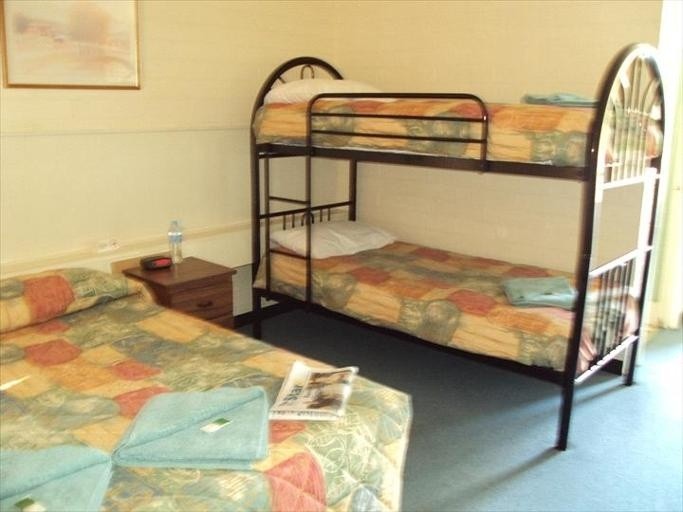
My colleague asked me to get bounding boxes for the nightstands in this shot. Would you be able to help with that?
[121,256,237,331]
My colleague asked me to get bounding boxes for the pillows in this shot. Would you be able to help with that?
[263,78,398,103]
[0,267,143,333]
[269,219,396,259]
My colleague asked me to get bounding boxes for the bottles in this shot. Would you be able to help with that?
[166,217,184,264]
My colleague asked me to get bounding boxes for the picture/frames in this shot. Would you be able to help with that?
[0,0,141,91]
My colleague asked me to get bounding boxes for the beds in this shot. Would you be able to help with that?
[252,42,672,450]
[0,268,414,512]
